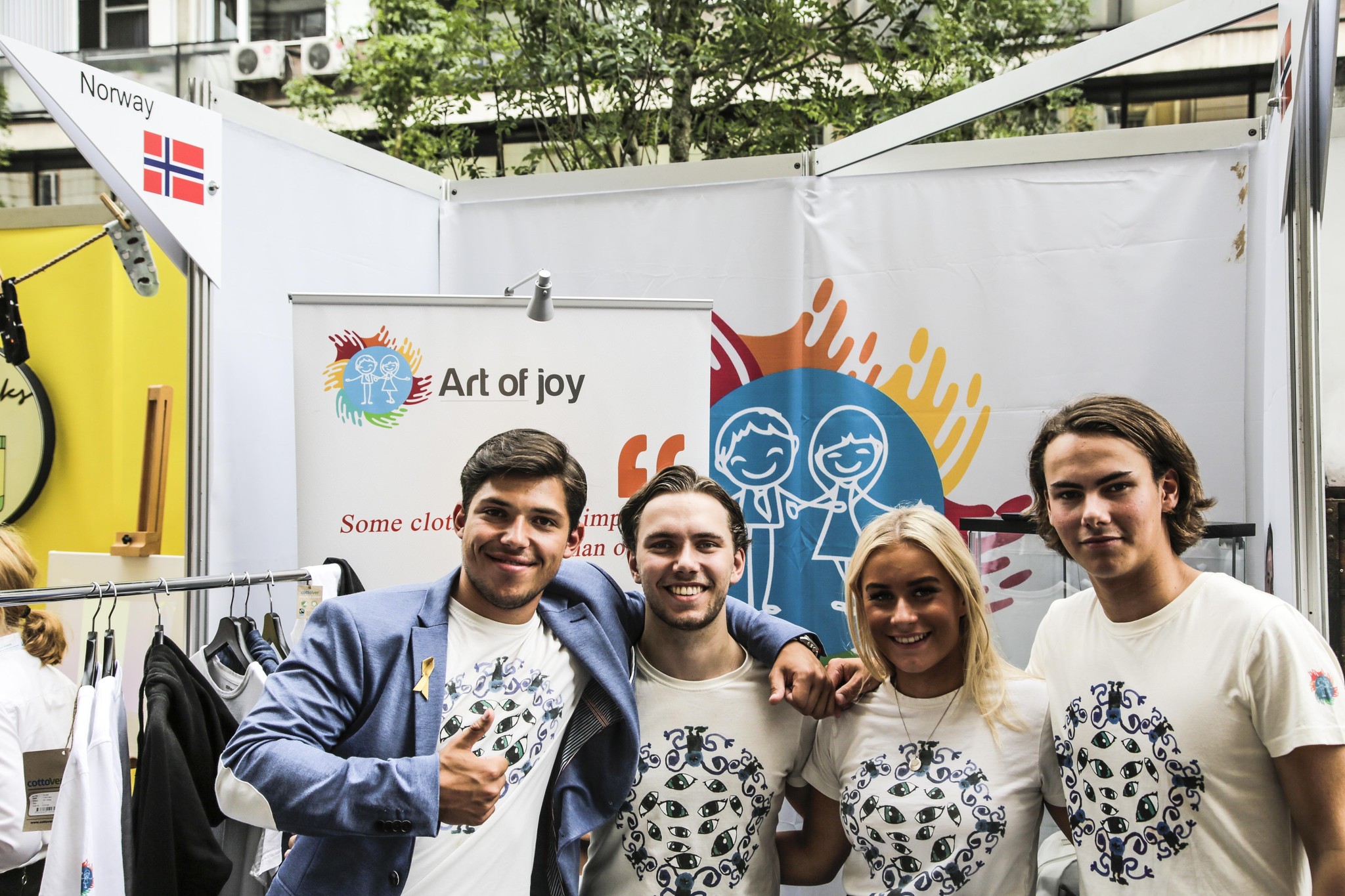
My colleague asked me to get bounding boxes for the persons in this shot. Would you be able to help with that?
[809,391,1345,896]
[0,517,93,896]
[763,503,1094,896]
[578,462,857,896]
[214,427,834,896]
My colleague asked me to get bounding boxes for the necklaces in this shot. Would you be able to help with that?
[888,668,977,774]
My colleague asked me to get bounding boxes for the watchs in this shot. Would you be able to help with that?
[779,629,822,661]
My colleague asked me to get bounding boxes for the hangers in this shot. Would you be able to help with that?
[80,581,104,690]
[236,571,257,639]
[98,581,119,681]
[202,571,255,671]
[152,576,170,646]
[259,569,292,660]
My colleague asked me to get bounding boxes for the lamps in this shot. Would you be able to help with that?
[503,268,556,322]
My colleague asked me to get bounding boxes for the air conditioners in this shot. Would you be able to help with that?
[299,34,356,76]
[229,38,286,82]
